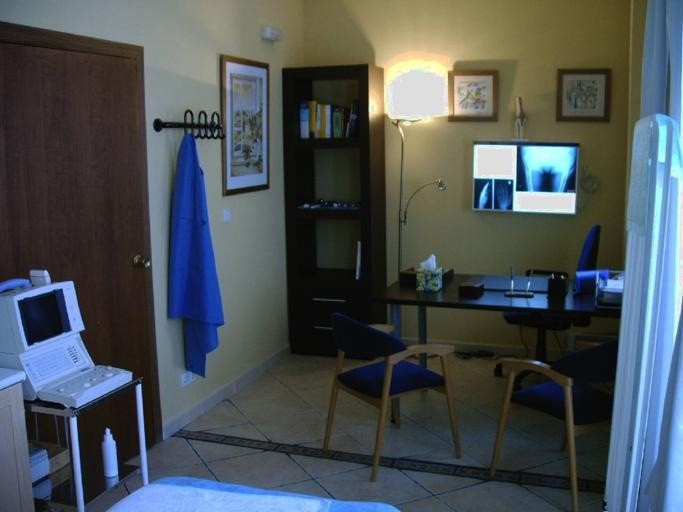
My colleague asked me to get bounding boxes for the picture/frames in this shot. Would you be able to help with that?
[557,68,611,122]
[447,71,498,122]
[220,52,269,197]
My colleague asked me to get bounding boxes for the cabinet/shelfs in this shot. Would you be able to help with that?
[0,366,35,512]
[281,65,386,359]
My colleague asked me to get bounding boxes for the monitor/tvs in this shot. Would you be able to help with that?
[14,280,92,352]
[472,141,580,217]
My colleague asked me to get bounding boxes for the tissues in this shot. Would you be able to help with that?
[416,253,443,295]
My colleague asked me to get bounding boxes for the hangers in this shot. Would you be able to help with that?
[152,109,223,140]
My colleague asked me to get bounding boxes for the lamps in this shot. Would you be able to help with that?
[384,57,448,359]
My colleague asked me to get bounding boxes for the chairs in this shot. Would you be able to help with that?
[323,311,462,483]
[493,225,602,377]
[491,338,618,512]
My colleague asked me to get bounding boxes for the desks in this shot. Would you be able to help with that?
[373,273,625,393]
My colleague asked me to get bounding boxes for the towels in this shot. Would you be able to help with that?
[167,133,226,379]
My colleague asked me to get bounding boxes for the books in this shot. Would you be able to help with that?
[300,100,356,139]
